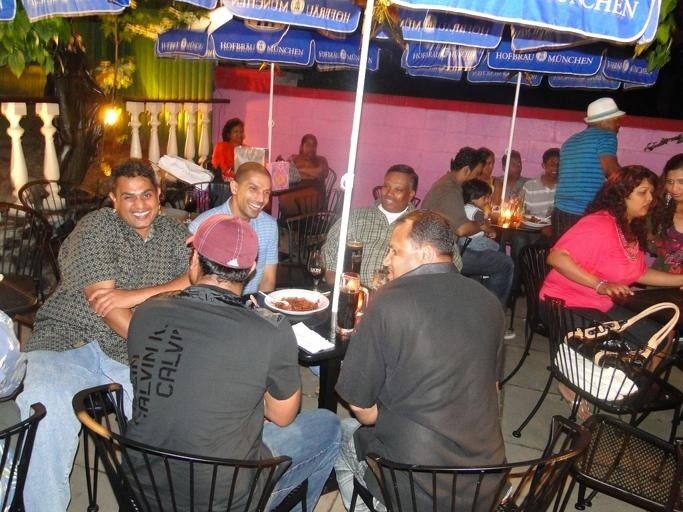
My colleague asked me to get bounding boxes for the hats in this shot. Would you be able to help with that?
[184,212,260,270]
[582,95,627,123]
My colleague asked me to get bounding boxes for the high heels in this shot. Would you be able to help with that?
[556,382,595,422]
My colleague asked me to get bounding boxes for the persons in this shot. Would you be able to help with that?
[0,311,27,509]
[211,118,245,180]
[540,154,682,417]
[424,144,560,338]
[289,134,329,250]
[124,217,341,512]
[322,164,463,288]
[13,158,192,509]
[189,160,280,296]
[333,209,509,512]
[550,98,626,242]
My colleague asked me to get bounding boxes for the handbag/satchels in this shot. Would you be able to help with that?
[233,145,267,174]
[267,160,293,193]
[561,299,683,380]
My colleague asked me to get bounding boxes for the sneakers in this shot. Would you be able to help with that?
[502,328,516,340]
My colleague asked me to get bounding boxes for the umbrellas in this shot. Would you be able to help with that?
[397,42,660,202]
[181,1,664,314]
[153,6,382,164]
[0,0,131,24]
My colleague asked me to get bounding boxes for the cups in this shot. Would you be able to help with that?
[371,270,393,297]
[306,249,327,294]
[342,239,363,275]
[336,272,361,334]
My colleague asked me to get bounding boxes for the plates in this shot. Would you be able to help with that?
[264,288,330,315]
[521,214,552,228]
